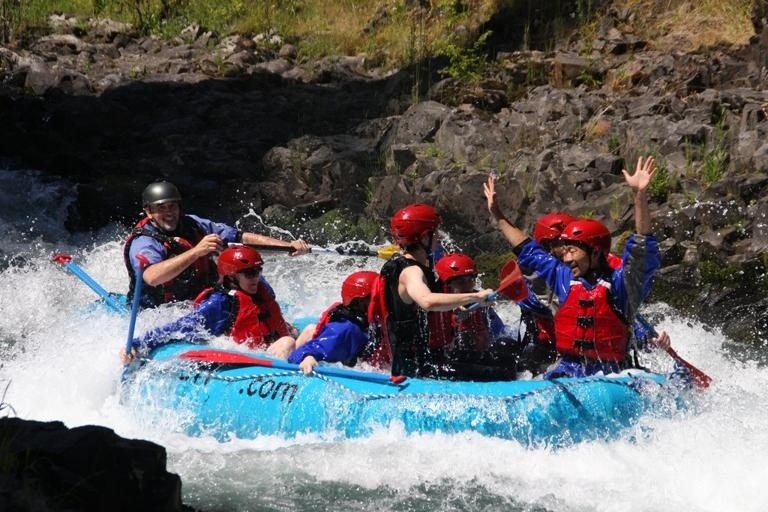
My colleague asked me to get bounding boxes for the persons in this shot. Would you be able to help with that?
[432,250,516,361]
[122,179,312,314]
[482,154,663,380]
[284,269,394,377]
[366,202,520,383]
[117,245,320,365]
[485,212,672,377]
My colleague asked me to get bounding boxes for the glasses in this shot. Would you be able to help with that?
[239,268,261,277]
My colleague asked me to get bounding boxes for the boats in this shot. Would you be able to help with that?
[50,294,687,450]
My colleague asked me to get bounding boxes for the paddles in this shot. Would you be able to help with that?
[457,260,529,321]
[180,351,407,384]
[636,313,714,390]
[220,243,402,262]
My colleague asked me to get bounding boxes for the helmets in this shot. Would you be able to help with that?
[391,204,440,247]
[142,181,184,217]
[535,213,611,269]
[341,271,379,306]
[435,254,477,292]
[216,245,263,284]
